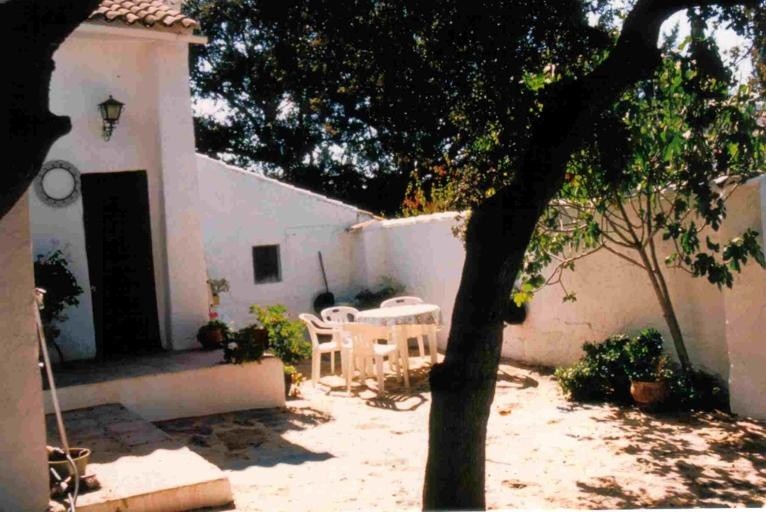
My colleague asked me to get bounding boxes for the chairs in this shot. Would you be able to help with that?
[298,296,438,398]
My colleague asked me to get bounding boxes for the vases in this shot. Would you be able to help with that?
[46,446,90,475]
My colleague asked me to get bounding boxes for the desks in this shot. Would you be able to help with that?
[357,304,440,382]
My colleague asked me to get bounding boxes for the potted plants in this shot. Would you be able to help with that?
[195,319,274,365]
[554,327,666,408]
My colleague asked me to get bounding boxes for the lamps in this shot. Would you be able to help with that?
[95,95,125,141]
